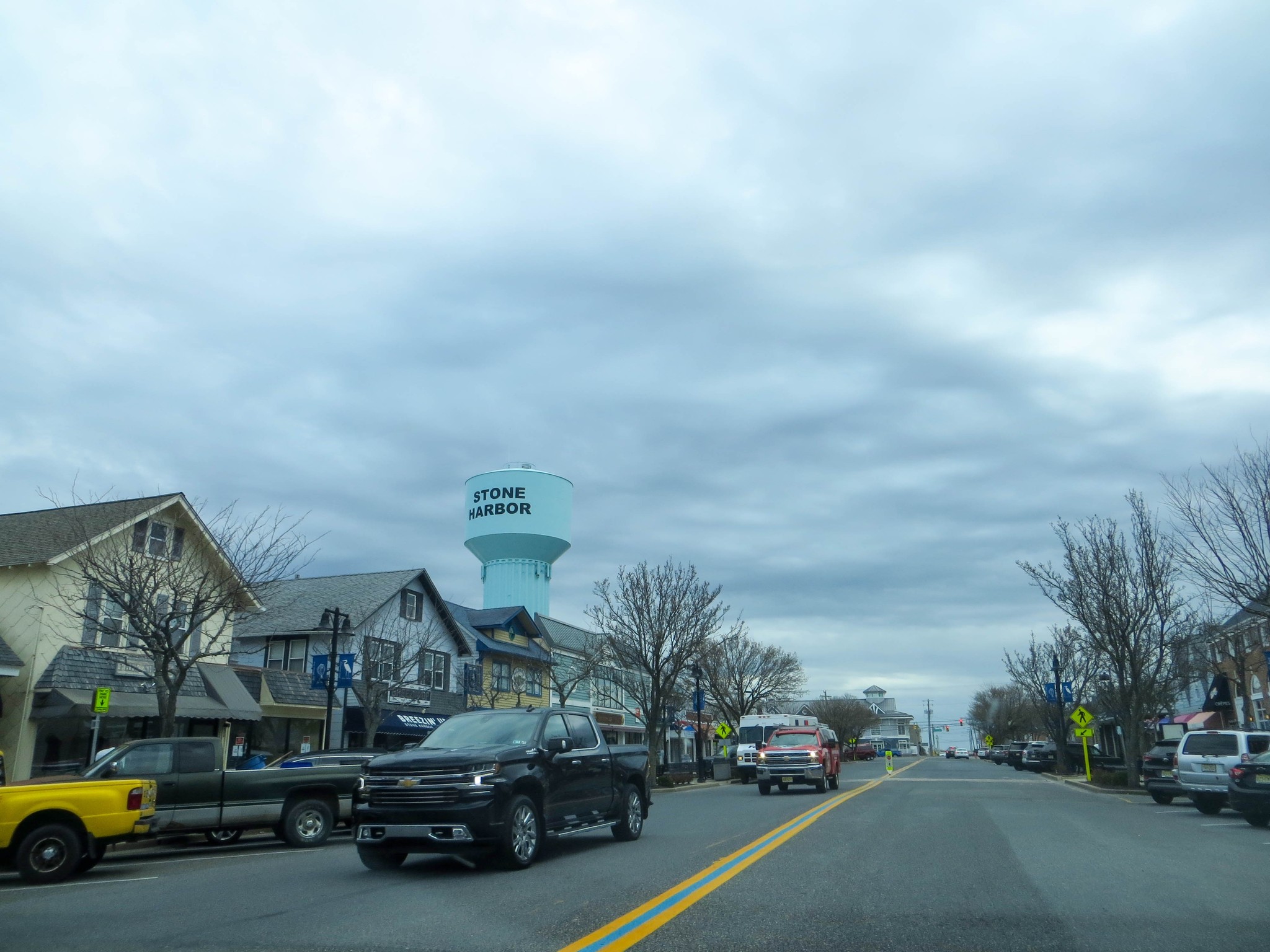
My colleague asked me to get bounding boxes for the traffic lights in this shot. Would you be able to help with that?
[960,719,963,726]
[946,726,949,732]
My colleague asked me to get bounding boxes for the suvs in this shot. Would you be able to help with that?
[977,747,990,760]
[1007,741,1058,774]
[946,746,959,758]
[1142,738,1198,805]
[990,745,1011,765]
[1171,728,1270,815]
[1040,740,1124,774]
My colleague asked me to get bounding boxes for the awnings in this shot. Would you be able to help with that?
[196,661,263,721]
[1202,672,1234,712]
[29,689,231,719]
[1157,712,1221,730]
[344,705,453,737]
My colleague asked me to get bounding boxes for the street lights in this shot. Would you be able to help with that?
[312,606,356,750]
[688,661,708,783]
[1049,649,1067,745]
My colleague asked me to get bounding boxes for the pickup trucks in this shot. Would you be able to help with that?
[875,749,903,757]
[754,723,841,795]
[72,736,364,886]
[350,705,653,873]
[0,745,157,888]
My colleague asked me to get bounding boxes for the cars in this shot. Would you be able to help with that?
[204,748,394,846]
[967,748,979,756]
[954,748,969,759]
[939,752,946,757]
[843,745,877,761]
[1228,749,1270,826]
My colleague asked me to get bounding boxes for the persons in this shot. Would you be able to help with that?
[1136,755,1144,777]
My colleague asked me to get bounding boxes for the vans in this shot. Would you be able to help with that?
[733,713,818,784]
